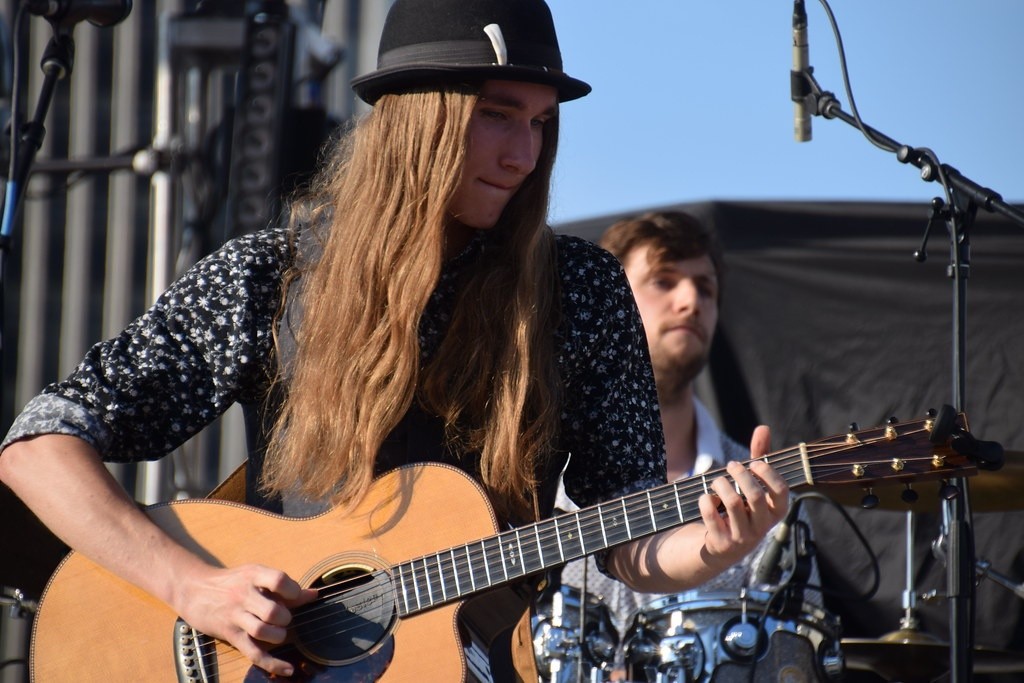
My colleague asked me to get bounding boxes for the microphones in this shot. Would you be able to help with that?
[792,0,814,143]
[21,0,134,28]
[757,499,804,585]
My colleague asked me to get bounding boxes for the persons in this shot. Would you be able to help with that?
[0,1,790,682]
[499,209,845,683]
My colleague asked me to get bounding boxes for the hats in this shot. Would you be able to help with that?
[350,0,593,103]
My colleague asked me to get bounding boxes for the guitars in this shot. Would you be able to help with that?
[28,403,1005,683]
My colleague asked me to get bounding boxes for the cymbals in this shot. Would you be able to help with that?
[784,439,1021,517]
[841,627,1006,682]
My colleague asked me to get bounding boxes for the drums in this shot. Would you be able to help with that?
[530,581,838,683]
